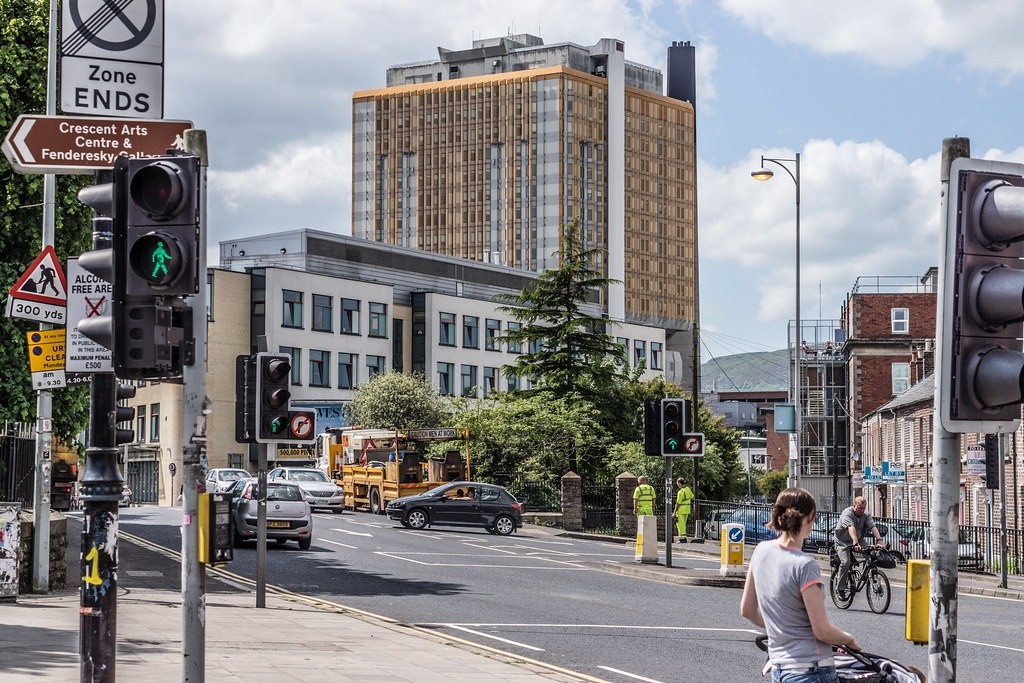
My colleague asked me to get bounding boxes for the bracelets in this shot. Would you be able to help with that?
[877,538,883,541]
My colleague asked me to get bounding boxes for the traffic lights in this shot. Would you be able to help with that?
[256,352,317,444]
[941,158,1024,434]
[116,382,136,447]
[662,399,705,457]
[126,156,198,296]
[978,434,998,489]
[78,156,145,381]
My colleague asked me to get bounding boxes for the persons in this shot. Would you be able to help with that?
[802,340,817,356]
[633,476,657,519]
[740,487,861,683]
[671,478,694,543]
[821,341,832,356]
[448,489,474,501]
[833,496,888,597]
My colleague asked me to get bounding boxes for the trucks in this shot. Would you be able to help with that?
[308,424,470,515]
[50,433,80,511]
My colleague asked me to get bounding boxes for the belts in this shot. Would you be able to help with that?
[762,656,835,676]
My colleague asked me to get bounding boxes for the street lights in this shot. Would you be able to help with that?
[751,153,801,489]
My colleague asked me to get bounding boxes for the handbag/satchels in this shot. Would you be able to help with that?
[870,547,896,569]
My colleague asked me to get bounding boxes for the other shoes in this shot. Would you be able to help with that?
[677,539,687,542]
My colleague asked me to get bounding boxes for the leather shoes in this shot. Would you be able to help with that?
[836,589,846,601]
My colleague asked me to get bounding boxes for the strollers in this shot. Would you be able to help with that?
[754,635,927,683]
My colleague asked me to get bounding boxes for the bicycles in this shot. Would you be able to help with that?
[830,541,892,614]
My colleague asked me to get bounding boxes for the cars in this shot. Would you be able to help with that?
[384,482,523,536]
[704,506,914,564]
[267,467,345,514]
[205,468,252,492]
[224,477,312,550]
[908,527,985,574]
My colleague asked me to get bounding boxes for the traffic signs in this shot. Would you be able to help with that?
[6,113,194,169]
